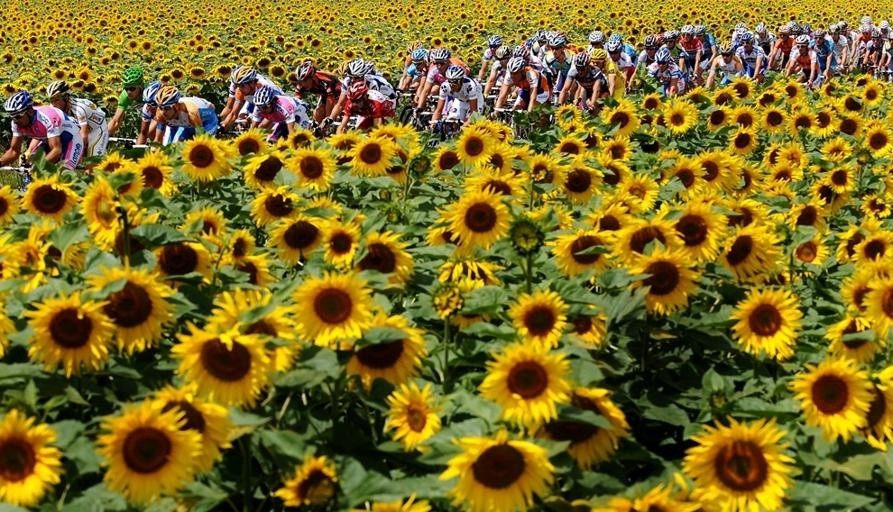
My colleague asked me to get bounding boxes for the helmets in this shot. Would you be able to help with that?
[4,92,33,111]
[407,42,464,84]
[296,61,313,82]
[253,85,273,107]
[155,85,180,106]
[143,83,162,103]
[46,81,68,99]
[337,60,374,103]
[121,67,144,87]
[231,65,256,85]
[488,17,893,73]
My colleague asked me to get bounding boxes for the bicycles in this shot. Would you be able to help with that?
[0,165,39,189]
[397,86,524,130]
[683,70,701,85]
[551,91,599,114]
[106,135,138,145]
[225,116,363,136]
[131,142,159,151]
[782,64,891,88]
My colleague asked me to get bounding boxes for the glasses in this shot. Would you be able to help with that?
[9,112,26,120]
[159,104,176,110]
[124,87,136,91]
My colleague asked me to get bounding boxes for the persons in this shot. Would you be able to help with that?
[396,47,487,127]
[2,92,83,170]
[295,59,399,133]
[477,13,892,110]
[108,66,221,149]
[217,64,321,142]
[47,80,109,156]
